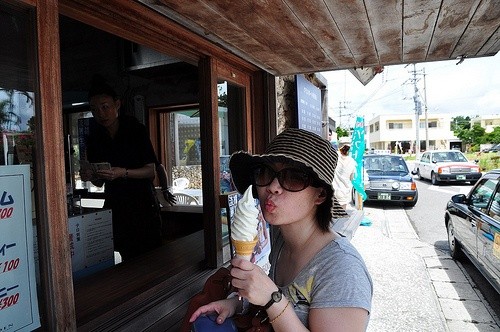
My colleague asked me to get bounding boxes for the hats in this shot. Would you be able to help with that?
[228,127,348,219]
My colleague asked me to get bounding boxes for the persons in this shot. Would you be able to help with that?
[79,82,161,249]
[190,128,373,332]
[391,160,403,170]
[332,136,357,205]
[156,163,177,206]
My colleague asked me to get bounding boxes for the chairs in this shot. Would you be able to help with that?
[172,177,200,205]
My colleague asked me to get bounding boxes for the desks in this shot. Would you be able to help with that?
[178,189,202,199]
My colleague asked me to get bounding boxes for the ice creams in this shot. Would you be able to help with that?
[230,185,259,258]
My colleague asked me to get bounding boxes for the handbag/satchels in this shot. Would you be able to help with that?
[235,303,271,332]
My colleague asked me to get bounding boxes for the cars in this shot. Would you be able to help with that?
[418,150,482,185]
[352,150,418,207]
[482,142,500,153]
[444,169,500,295]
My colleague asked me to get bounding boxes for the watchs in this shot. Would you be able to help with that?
[262,288,283,309]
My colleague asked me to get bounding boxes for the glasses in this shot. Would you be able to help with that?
[250,164,324,192]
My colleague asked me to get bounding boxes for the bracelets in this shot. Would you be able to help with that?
[270,301,290,323]
[125,168,128,176]
